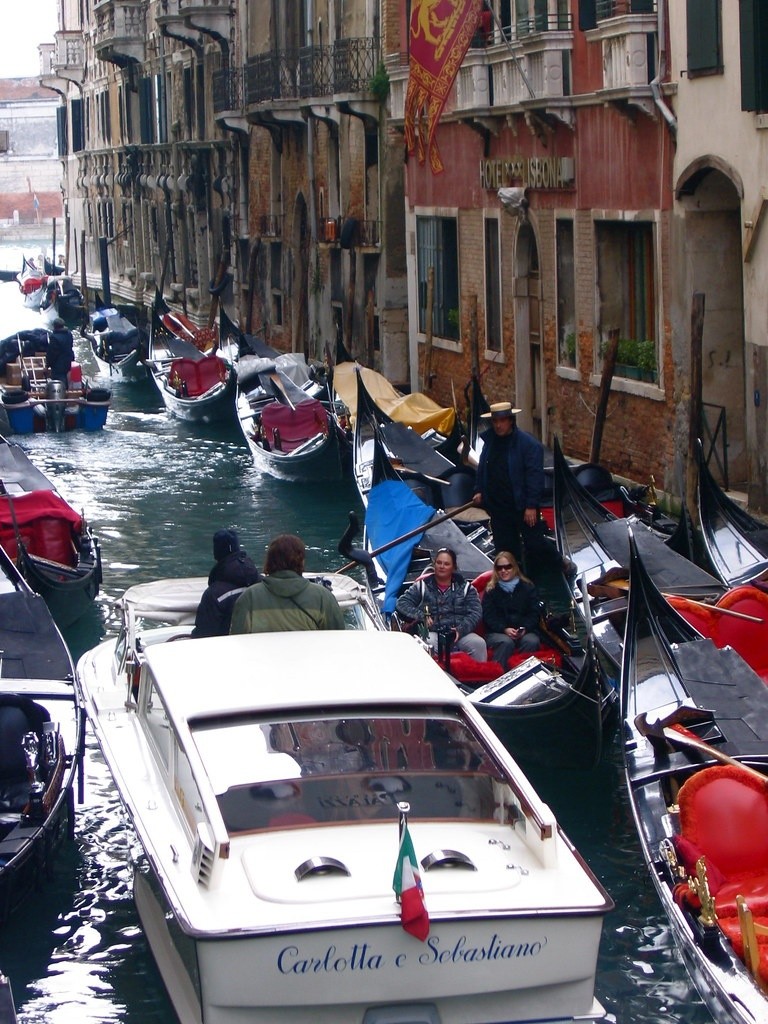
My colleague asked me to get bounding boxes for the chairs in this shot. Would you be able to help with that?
[714,585,768,689]
[666,595,713,639]
[261,398,329,452]
[540,472,556,510]
[412,569,567,679]
[0,517,74,580]
[575,463,623,503]
[169,354,227,397]
[669,765,768,1002]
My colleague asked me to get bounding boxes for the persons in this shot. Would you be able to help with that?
[43,317,75,391]
[190,531,265,640]
[396,548,488,663]
[29,255,66,271]
[473,402,544,588]
[0,693,51,813]
[481,552,540,673]
[228,535,345,636]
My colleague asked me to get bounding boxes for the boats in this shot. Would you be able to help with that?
[0,328,113,435]
[219,300,337,402]
[324,325,455,446]
[38,274,86,329]
[0,431,104,601]
[42,255,66,275]
[76,571,617,1024]
[351,364,618,747]
[230,330,348,483]
[88,289,139,376]
[155,286,221,359]
[146,301,233,422]
[551,431,768,706]
[617,525,768,1024]
[0,544,87,909]
[15,255,47,296]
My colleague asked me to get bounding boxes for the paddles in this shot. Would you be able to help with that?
[167,314,196,339]
[135,356,184,367]
[270,374,296,412]
[334,500,476,574]
[662,726,768,783]
[391,463,451,485]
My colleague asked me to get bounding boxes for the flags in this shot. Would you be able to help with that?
[392,821,431,944]
[33,191,39,210]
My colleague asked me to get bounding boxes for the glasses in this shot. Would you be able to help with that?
[495,563,513,571]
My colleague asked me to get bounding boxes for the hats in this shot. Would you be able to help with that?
[213,530,241,559]
[480,402,523,419]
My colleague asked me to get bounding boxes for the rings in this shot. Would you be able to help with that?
[509,634,512,636]
